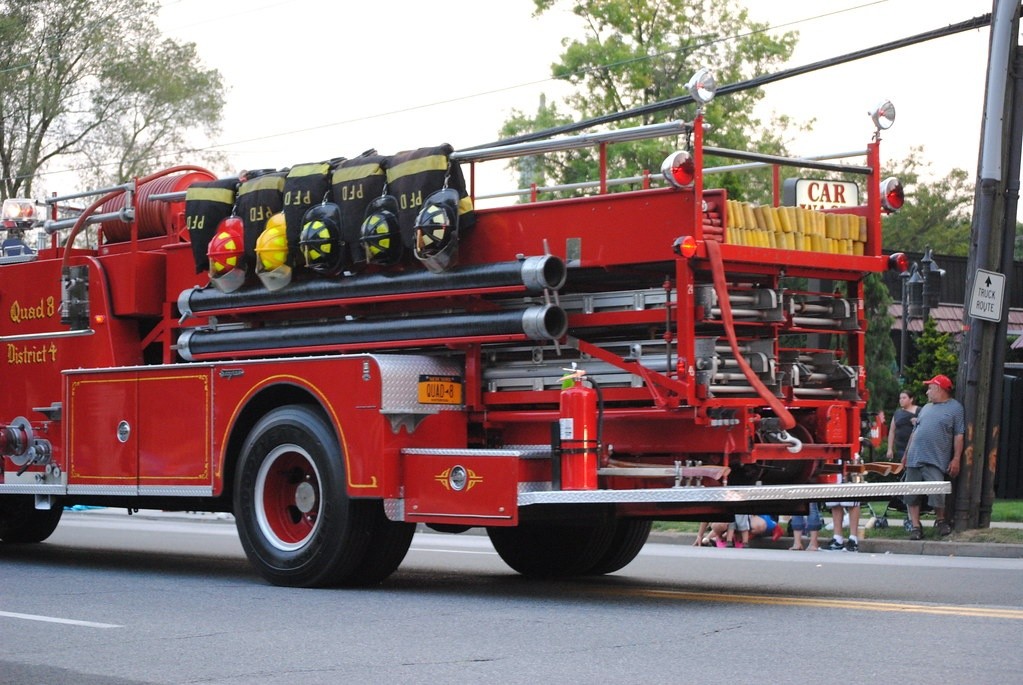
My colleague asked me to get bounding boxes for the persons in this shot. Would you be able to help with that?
[900,375,965,541]
[886,390,922,481]
[692,452,860,553]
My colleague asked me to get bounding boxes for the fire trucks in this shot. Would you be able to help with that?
[0,67,954,591]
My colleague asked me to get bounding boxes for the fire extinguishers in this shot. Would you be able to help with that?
[557,361,604,490]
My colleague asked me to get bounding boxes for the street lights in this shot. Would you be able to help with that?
[897,261,923,377]
[920,244,946,333]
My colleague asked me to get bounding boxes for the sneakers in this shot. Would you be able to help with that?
[841,539,859,553]
[909,521,925,540]
[934,519,951,537]
[817,538,847,552]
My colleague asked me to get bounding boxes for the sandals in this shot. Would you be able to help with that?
[805,543,820,552]
[788,545,805,550]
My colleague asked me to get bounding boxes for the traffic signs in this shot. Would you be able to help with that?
[968,268,1007,323]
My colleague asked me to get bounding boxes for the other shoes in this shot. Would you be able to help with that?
[771,525,784,541]
[748,532,758,540]
[742,542,751,548]
[726,541,733,548]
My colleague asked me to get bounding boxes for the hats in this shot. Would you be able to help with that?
[922,374,952,392]
